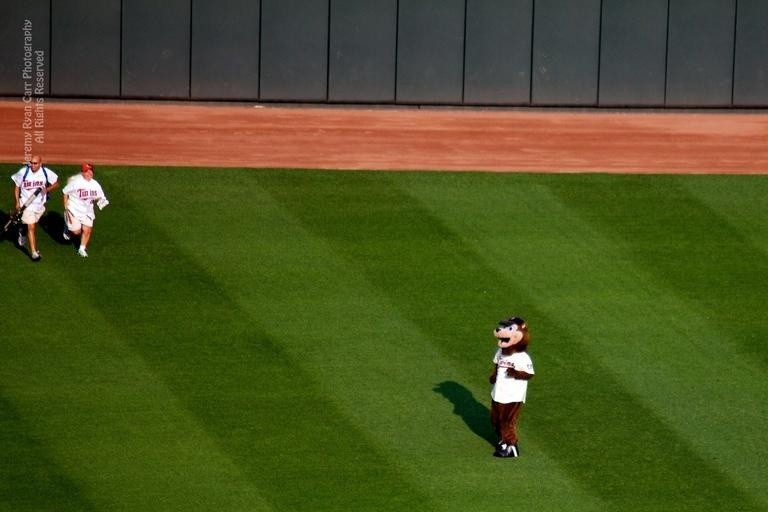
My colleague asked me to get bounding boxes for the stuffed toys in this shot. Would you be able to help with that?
[490,316,536,458]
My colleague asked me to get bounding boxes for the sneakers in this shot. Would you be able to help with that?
[18,231,26,246]
[32,251,40,260]
[76,248,89,258]
[63,226,71,241]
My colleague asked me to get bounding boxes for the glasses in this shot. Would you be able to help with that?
[29,161,38,164]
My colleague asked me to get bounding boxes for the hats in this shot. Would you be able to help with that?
[81,163,91,172]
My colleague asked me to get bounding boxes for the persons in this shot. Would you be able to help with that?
[61,162,109,258]
[10,155,60,262]
